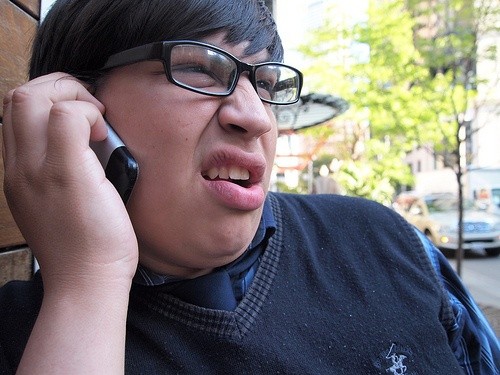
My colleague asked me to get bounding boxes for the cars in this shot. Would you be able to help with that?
[406,193,500,258]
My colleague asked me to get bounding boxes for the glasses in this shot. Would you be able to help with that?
[90,40,303,106]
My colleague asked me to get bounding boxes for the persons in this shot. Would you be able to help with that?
[0,0,500,375]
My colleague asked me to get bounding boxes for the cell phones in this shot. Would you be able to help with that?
[89,117,139,208]
